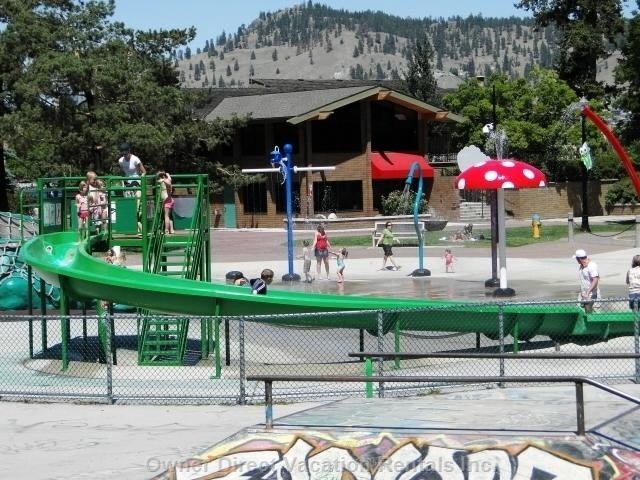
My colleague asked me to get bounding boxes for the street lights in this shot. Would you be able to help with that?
[577,95,593,233]
[475,74,497,159]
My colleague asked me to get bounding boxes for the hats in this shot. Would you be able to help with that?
[573,250,586,258]
[120,143,129,155]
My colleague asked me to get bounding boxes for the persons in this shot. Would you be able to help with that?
[296,240,316,283]
[250,267,274,296]
[441,247,459,273]
[571,249,603,313]
[373,220,405,272]
[625,254,640,312]
[118,142,146,183]
[328,247,349,284]
[104,249,117,264]
[75,169,175,236]
[113,252,126,267]
[311,223,334,281]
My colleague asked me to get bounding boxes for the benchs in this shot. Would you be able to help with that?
[371,222,425,249]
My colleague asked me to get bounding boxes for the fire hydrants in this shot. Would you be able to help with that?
[531,213,543,239]
